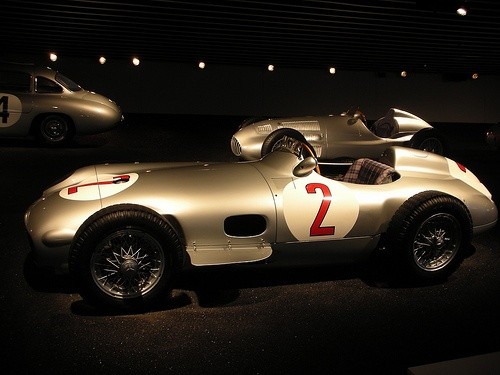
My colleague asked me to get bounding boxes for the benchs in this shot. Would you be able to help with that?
[343,157,395,186]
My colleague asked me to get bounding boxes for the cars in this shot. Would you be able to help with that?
[2,62,127,149]
[25,149,498,320]
[230,106,447,170]
